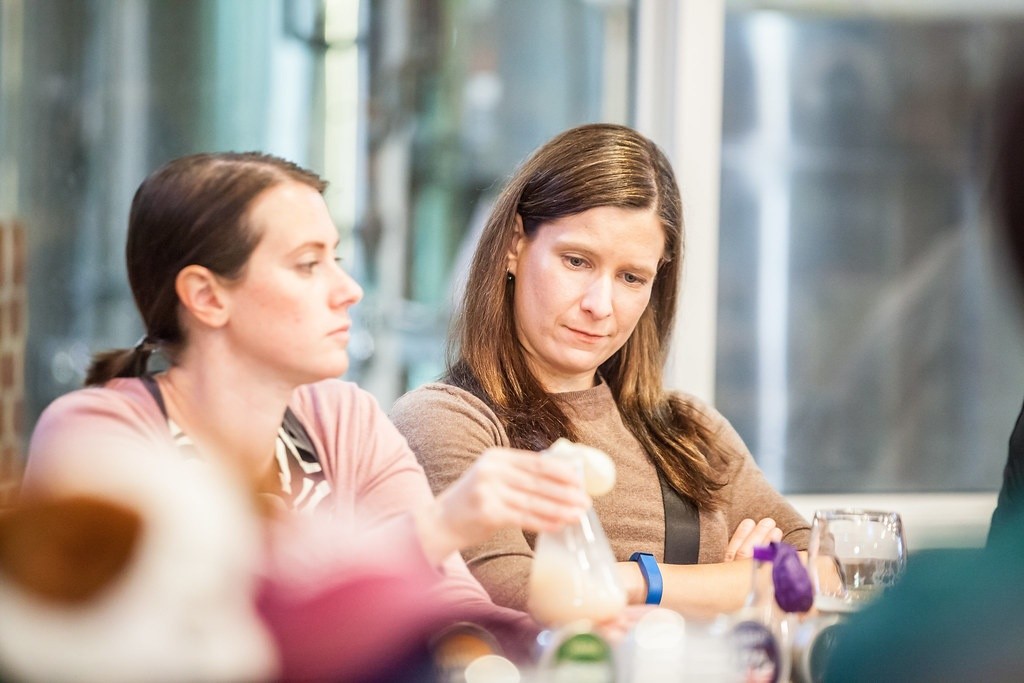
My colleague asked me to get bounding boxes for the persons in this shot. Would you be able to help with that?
[773,34,1024,682]
[11,150,589,683]
[387,121,845,628]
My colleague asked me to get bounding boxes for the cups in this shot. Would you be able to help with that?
[810,507,909,626]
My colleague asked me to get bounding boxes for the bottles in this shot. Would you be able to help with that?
[523,449,630,624]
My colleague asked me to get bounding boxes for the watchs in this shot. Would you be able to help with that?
[629,552,662,605]
